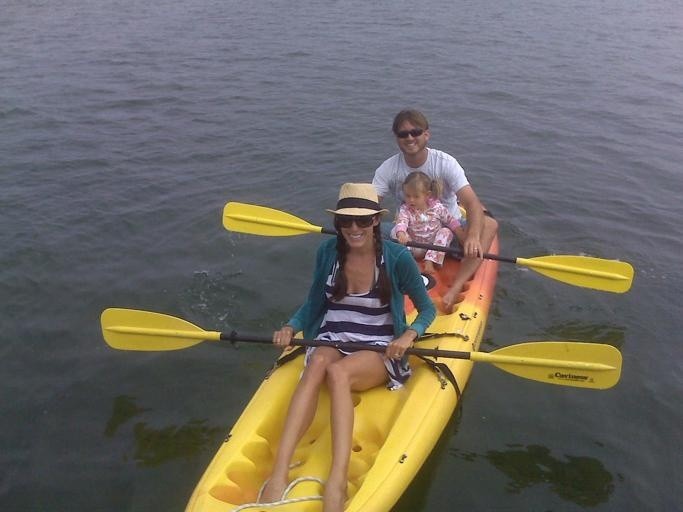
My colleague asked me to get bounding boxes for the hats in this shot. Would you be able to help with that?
[325,183,390,216]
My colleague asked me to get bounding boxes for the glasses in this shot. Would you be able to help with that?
[397,128,423,138]
[336,216,373,228]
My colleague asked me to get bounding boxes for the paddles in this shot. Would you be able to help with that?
[221,201,634,294]
[100,308,622,391]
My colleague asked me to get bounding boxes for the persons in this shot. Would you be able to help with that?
[389,170,468,276]
[257,181,436,512]
[371,109,498,315]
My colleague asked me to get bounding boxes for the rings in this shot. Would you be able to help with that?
[393,354,398,356]
[473,248,477,250]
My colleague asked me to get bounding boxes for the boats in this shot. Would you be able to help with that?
[182,200,500,512]
